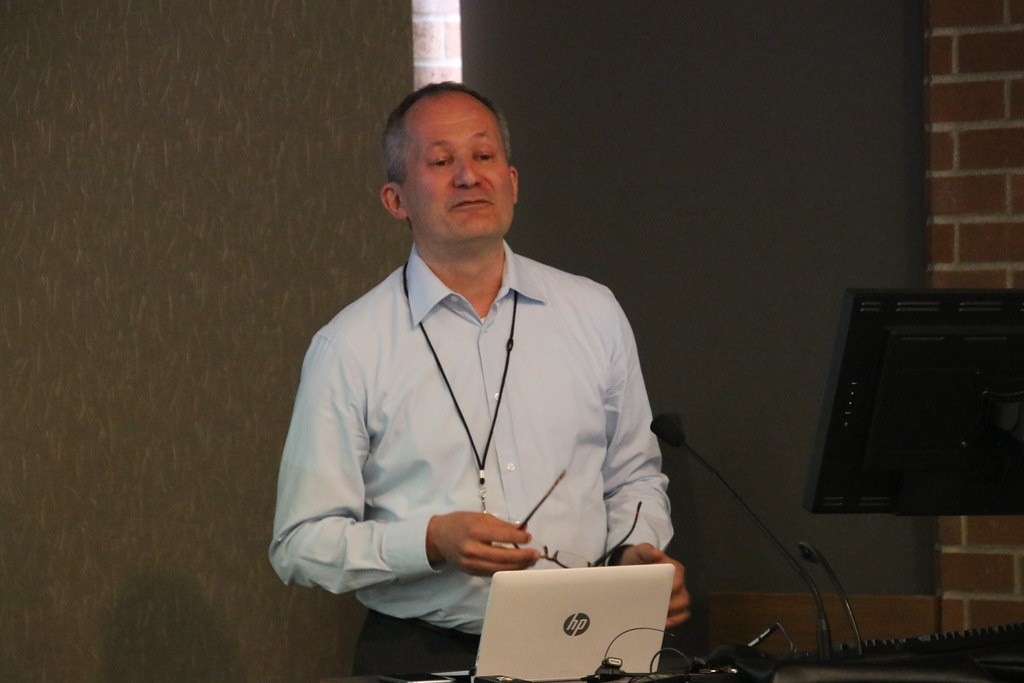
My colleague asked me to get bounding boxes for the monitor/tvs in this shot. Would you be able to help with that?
[803,286,1024,516]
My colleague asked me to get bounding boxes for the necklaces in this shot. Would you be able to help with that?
[398,259,518,512]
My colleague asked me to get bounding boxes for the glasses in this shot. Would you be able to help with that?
[514,470,643,568]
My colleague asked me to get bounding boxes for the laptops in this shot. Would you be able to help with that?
[429,564,675,683]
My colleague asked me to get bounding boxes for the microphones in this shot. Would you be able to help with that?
[650,413,831,662]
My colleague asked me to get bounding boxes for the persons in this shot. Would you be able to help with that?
[268,82,692,682]
